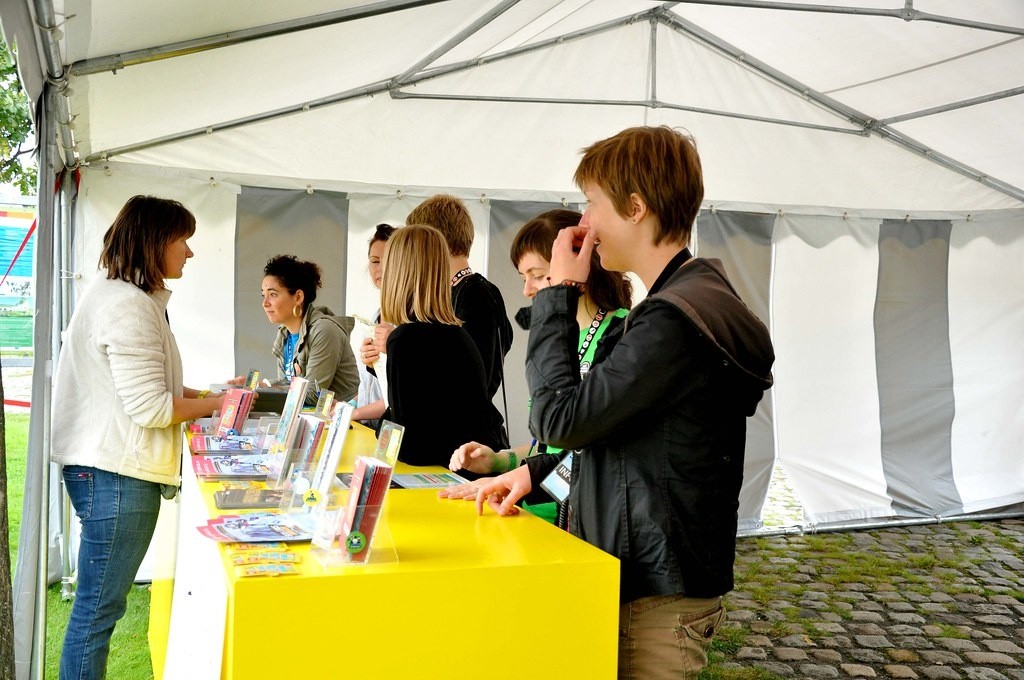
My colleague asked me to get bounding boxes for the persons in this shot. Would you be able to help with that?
[226,255,359,414]
[438,210,629,530]
[473,128,773,680]
[398,194,514,458]
[47,197,259,680]
[352,222,399,426]
[375,224,503,480]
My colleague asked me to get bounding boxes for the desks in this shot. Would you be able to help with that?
[146,408,621,680]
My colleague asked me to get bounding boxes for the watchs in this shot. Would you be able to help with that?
[499,449,517,470]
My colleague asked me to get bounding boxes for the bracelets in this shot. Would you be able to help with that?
[197,390,213,401]
[558,280,586,294]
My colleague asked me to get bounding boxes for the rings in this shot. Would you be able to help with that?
[493,491,498,496]
[362,352,368,358]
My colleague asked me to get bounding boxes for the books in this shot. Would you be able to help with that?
[184,378,393,570]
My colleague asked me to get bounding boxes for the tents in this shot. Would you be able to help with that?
[1,0,1023,680]
